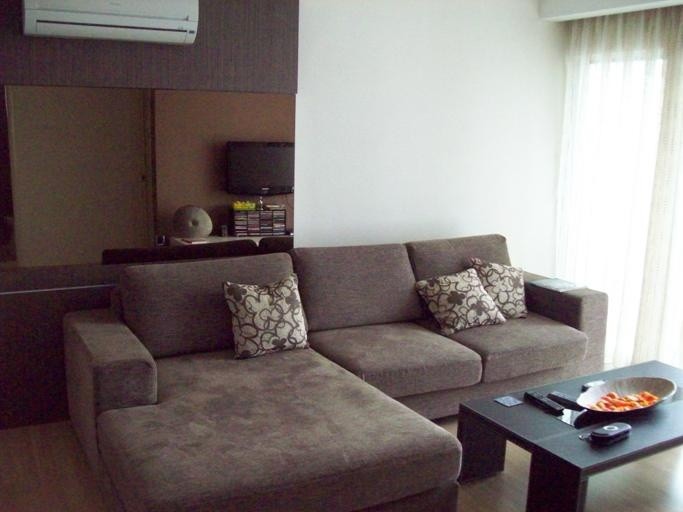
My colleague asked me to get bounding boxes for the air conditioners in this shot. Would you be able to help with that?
[22,0,200,45]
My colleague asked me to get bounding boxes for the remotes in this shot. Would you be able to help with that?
[548,391,586,410]
[526,391,564,413]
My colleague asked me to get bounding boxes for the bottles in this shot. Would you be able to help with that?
[256,196,264,211]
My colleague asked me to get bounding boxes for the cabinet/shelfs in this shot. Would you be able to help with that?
[228,208,286,237]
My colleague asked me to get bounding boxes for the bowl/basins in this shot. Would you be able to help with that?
[574,375,677,413]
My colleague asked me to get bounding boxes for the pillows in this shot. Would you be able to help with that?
[220,272,309,361]
[468,251,527,318]
[415,267,505,335]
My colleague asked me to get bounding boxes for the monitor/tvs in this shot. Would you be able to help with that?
[224,141,294,196]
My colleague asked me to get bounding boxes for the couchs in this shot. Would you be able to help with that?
[61,233,610,512]
